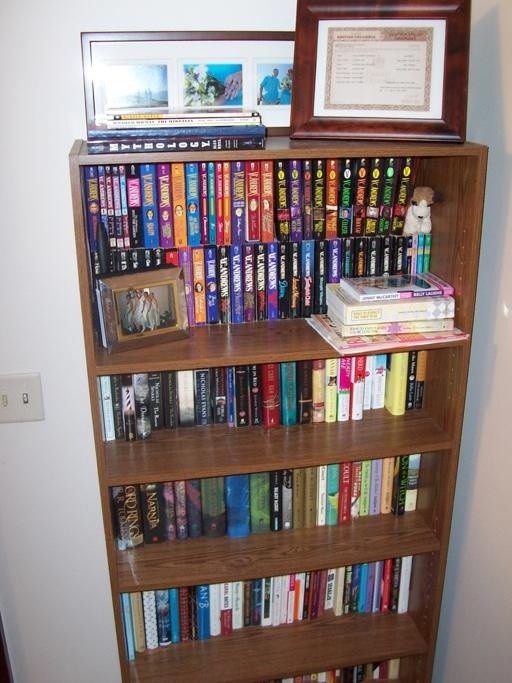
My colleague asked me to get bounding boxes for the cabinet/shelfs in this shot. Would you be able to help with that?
[68,140,488,683]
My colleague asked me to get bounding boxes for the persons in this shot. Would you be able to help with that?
[259,67,292,105]
[184,65,243,105]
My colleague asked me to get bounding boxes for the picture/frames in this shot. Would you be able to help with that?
[289,0,471,143]
[79,31,296,140]
[95,264,191,356]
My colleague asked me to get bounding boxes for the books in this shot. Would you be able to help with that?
[109,454,421,549]
[100,349,427,441]
[84,158,468,354]
[273,658,400,682]
[87,109,267,153]
[122,555,413,660]
